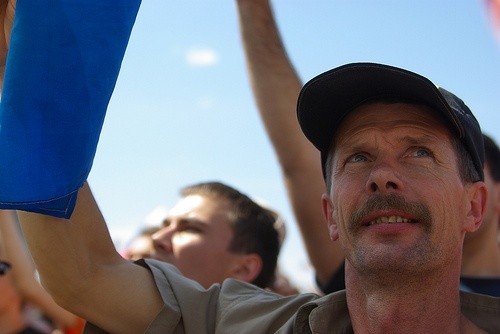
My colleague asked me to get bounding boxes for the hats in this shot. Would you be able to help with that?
[296,63,485,181]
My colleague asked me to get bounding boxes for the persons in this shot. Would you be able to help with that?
[0,257,52,334]
[237,0,500,298]
[0,1,500,334]
[0,209,160,334]
[0,182,279,333]
